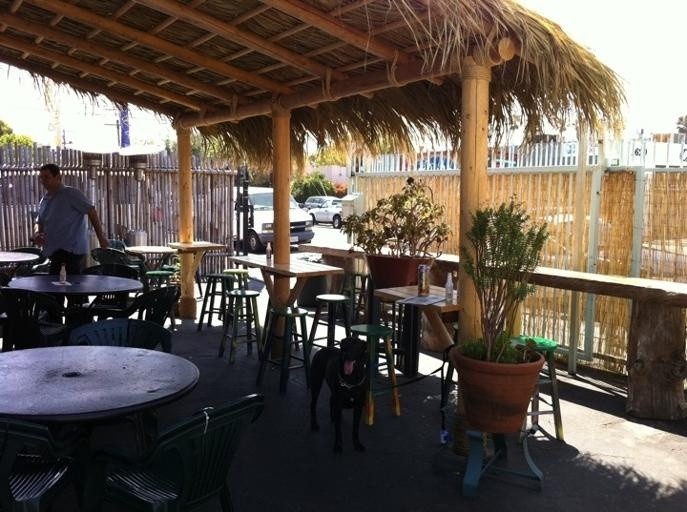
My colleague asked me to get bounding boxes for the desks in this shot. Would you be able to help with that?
[168,241,227,319]
[374,283,461,353]
[0,345,200,458]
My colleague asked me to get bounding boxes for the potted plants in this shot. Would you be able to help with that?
[449,193,546,431]
[340,178,452,302]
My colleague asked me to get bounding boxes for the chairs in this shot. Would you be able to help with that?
[105,391,265,512]
[0,239,181,353]
[0,418,86,512]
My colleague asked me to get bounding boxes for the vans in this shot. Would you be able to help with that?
[204,184,311,253]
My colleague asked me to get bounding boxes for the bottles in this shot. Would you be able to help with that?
[59,262,67,284]
[417,264,430,297]
[265,240,272,259]
[444,272,454,304]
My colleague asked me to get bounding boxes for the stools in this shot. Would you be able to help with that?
[198,269,401,425]
[510,337,564,442]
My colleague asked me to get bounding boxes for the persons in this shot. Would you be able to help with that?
[33,164,110,324]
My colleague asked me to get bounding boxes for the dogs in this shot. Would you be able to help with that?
[309,337,369,457]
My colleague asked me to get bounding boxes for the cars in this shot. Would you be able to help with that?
[304,196,348,225]
